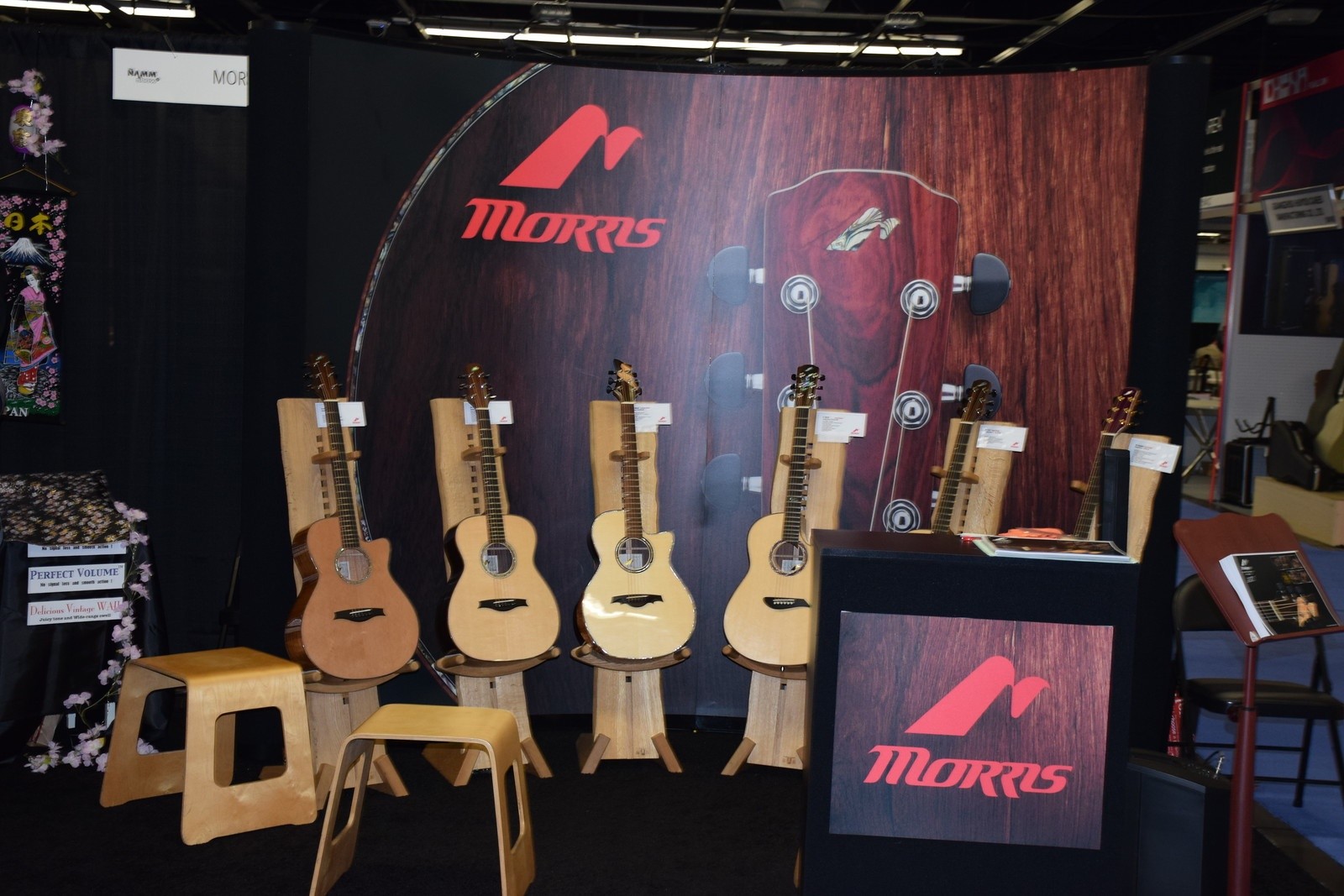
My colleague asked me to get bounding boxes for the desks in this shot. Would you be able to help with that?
[1182,400,1220,482]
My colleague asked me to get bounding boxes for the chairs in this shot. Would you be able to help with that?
[1171,577,1344,806]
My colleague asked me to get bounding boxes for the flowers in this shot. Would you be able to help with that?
[26,502,164,778]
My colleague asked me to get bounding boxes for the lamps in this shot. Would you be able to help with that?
[886,11,927,28]
[531,2,572,21]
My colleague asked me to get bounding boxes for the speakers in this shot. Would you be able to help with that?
[1124,747,1232,896]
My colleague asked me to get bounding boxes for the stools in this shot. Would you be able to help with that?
[100,647,320,847]
[309,704,535,896]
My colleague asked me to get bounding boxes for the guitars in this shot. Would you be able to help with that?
[284,350,421,680]
[576,363,698,665]
[1073,387,1140,540]
[723,364,825,667]
[438,362,562,662]
[931,379,997,534]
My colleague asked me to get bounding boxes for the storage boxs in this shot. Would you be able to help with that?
[1253,476,1344,546]
